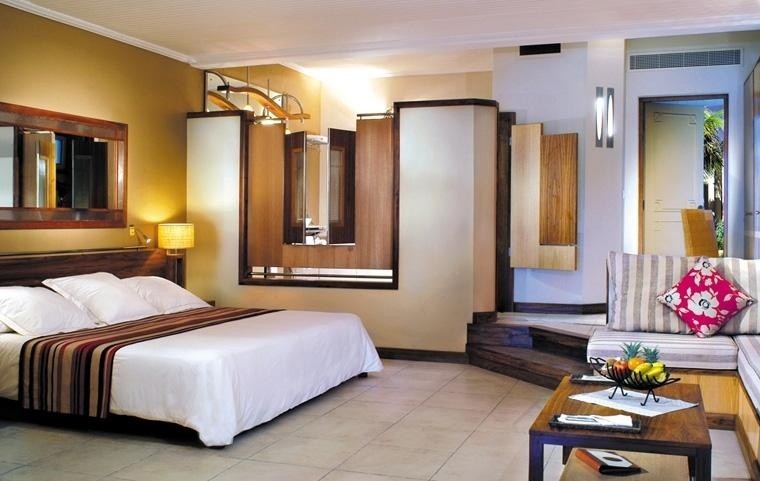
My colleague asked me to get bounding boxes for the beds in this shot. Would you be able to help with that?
[1,249,385,450]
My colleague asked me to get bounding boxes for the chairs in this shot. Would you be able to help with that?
[682,206,719,258]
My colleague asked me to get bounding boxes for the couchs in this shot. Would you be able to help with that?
[586,249,760,480]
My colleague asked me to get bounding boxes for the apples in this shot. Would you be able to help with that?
[613,361,628,375]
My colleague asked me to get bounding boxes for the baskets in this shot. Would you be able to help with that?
[590,355,680,391]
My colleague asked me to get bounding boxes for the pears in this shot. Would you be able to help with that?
[628,357,644,371]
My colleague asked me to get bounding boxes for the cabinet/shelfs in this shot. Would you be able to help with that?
[743,53,760,260]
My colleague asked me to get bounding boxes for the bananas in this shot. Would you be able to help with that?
[633,363,652,375]
[652,363,665,368]
[642,367,665,382]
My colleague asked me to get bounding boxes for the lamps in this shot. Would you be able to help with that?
[593,81,616,151]
[157,222,197,284]
[135,228,151,246]
[239,64,293,137]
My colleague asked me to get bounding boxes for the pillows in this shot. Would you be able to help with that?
[656,255,756,342]
[116,275,214,316]
[1,285,99,337]
[41,270,159,327]
